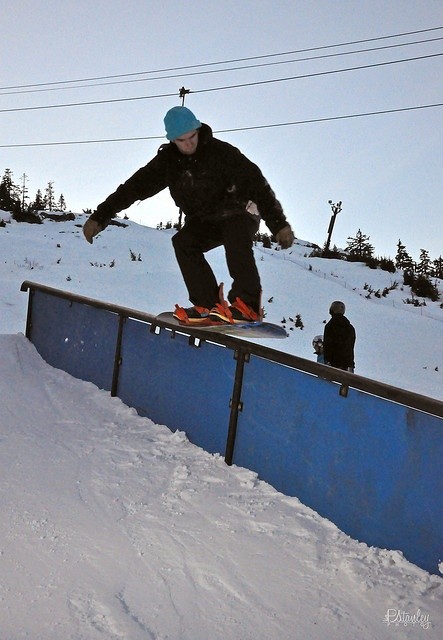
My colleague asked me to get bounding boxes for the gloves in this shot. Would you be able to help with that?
[275,225,296,250]
[82,218,102,244]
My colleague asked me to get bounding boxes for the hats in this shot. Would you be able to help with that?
[164,106,202,140]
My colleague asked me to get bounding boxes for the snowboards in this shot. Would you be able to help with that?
[153,312,287,339]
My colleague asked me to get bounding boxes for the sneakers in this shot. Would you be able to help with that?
[173,305,210,322]
[210,297,257,324]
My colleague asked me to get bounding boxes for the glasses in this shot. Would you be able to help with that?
[312,340,322,349]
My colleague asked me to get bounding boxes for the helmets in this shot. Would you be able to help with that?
[330,301,345,315]
[313,335,324,344]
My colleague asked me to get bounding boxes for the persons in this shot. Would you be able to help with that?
[322,301,356,374]
[84,107,295,326]
[312,335,324,364]
